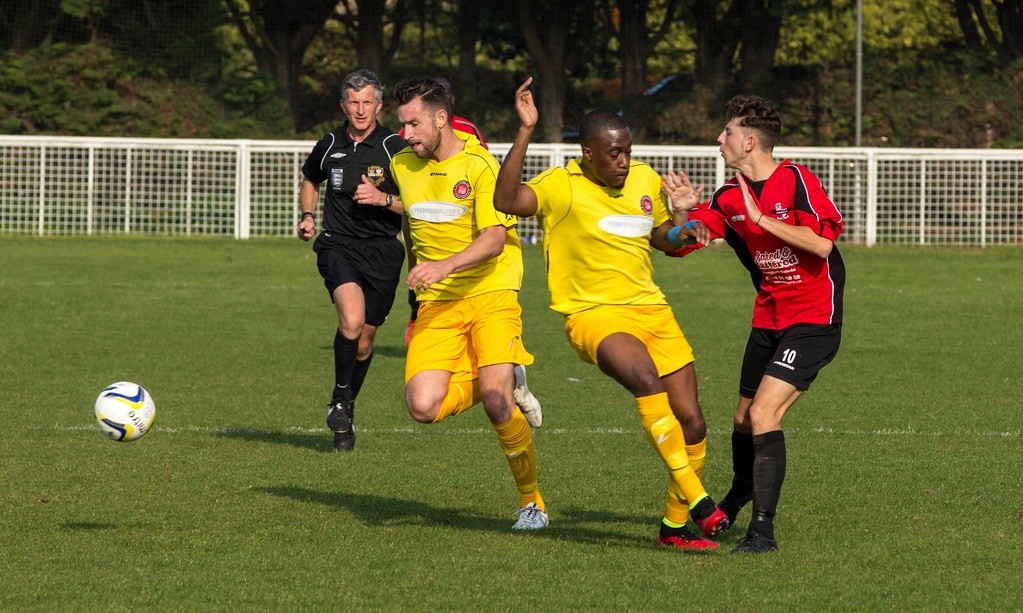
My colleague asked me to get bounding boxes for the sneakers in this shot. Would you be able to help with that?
[511,504,549,530]
[716,501,735,530]
[690,496,729,537]
[326,397,349,433]
[731,532,779,554]
[656,521,719,550]
[334,418,355,451]
[512,363,544,429]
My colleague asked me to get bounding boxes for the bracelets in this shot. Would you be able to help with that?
[302,212,314,219]
[756,214,763,225]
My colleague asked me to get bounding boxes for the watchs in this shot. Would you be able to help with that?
[385,192,394,207]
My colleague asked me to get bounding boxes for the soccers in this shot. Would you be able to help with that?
[94,380,157,443]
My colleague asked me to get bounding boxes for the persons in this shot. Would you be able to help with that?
[388,78,549,531]
[493,77,729,552]
[662,94,846,555]
[297,68,405,452]
[398,77,489,346]
